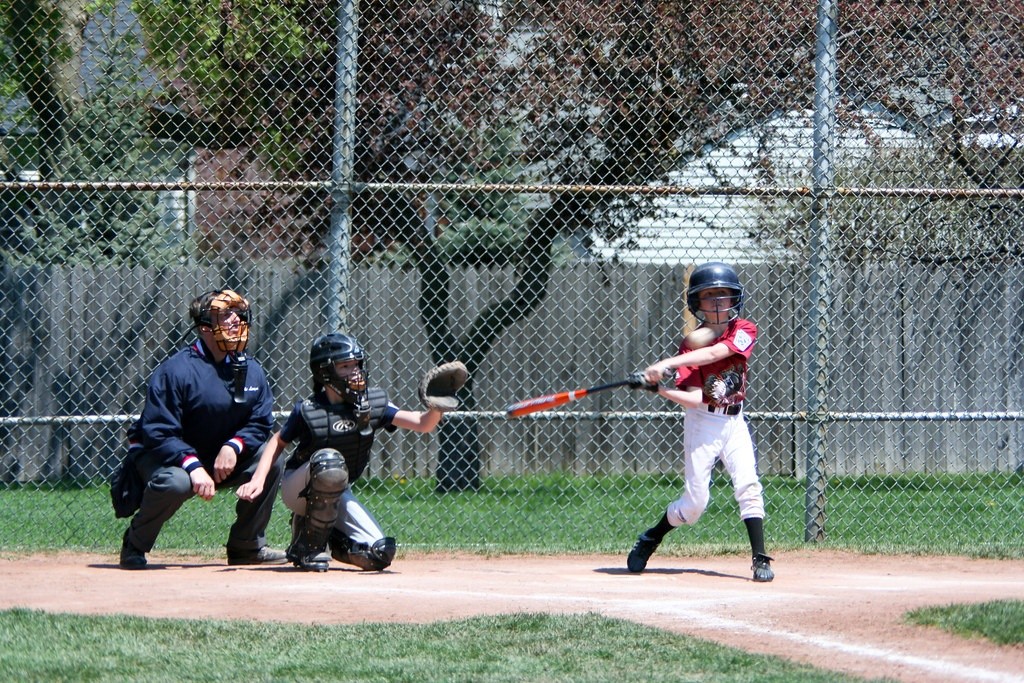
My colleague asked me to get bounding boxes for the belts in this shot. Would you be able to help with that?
[709,403,742,415]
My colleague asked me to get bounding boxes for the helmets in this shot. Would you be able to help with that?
[687,262,745,325]
[310,332,371,405]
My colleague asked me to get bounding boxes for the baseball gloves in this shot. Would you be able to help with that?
[417,361,468,411]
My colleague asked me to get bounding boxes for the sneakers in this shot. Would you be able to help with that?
[286,548,329,572]
[627,528,663,573]
[288,511,304,543]
[227,546,289,565]
[750,552,775,582]
[120,527,146,568]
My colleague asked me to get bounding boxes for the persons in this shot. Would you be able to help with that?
[235,333,468,572]
[626,261,775,582]
[109,289,288,569]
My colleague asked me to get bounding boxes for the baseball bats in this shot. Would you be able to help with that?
[506,369,673,418]
